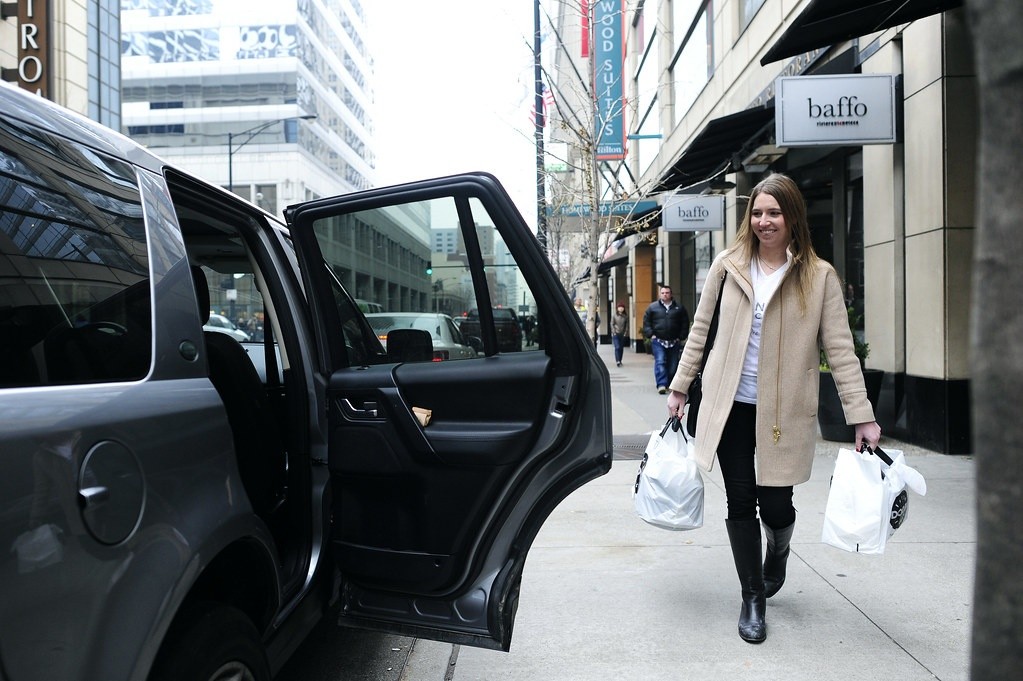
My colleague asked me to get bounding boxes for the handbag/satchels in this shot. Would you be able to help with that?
[630,414,706,531]
[686,372,702,437]
[820,442,927,555]
[622,334,631,347]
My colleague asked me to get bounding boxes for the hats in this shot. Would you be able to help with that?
[617,301,625,307]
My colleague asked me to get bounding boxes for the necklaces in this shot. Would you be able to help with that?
[759,257,777,271]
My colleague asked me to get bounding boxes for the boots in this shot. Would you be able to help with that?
[725,518,767,642]
[759,508,797,599]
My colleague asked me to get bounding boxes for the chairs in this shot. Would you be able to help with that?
[191,264,288,446]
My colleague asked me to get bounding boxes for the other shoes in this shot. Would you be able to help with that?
[617,361,621,366]
[658,387,666,394]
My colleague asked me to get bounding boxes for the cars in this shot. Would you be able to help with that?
[363,311,480,363]
[203,312,252,343]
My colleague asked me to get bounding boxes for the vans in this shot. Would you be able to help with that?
[354,298,383,317]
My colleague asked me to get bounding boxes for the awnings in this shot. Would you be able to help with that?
[598,246,629,274]
[646,48,855,198]
[576,268,590,284]
[614,205,662,241]
[760,0,964,67]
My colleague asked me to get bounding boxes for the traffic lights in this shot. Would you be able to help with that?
[426,261,432,275]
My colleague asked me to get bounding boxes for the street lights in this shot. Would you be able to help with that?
[229,115,316,196]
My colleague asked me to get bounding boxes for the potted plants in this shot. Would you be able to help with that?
[818,303,884,443]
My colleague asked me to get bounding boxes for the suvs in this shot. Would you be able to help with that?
[0,80,615,681]
[459,308,523,353]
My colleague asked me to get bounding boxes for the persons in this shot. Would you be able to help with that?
[667,174,881,644]
[643,286,690,393]
[574,298,601,351]
[612,302,629,366]
[526,315,535,347]
[248,311,264,342]
